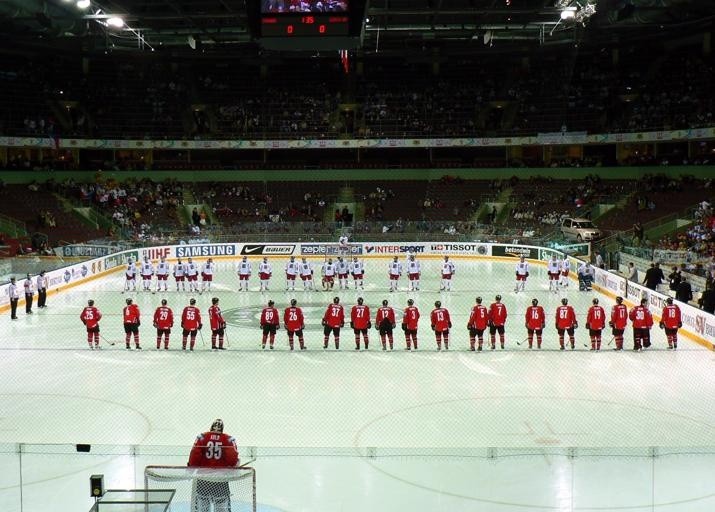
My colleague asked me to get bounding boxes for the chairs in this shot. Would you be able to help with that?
[0,86,715,275]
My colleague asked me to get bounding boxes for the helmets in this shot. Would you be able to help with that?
[476,297,482,303]
[408,299,414,305]
[358,298,363,305]
[88,300,94,304]
[190,299,196,304]
[434,301,441,306]
[532,299,538,306]
[383,300,388,306]
[334,297,339,303]
[641,299,647,306]
[268,300,275,306]
[162,300,166,305]
[496,296,501,300]
[562,298,568,305]
[667,298,673,304]
[593,298,599,304]
[212,298,218,305]
[126,298,132,303]
[291,299,297,306]
[211,419,223,432]
[616,297,623,303]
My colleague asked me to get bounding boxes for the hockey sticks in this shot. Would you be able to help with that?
[99,334,114,345]
[121,277,127,294]
[151,276,157,294]
[583,341,591,347]
[515,276,527,293]
[517,338,529,345]
[311,273,319,291]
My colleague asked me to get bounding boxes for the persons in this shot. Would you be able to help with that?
[375,300,396,350]
[237,256,252,292]
[180,298,202,350]
[406,254,421,290]
[660,297,682,349]
[0,85,715,244]
[80,300,102,348]
[578,261,595,291]
[260,300,280,350]
[284,256,314,291]
[188,419,241,512]
[259,257,272,291]
[431,301,452,350]
[628,262,715,315]
[284,299,308,350]
[208,298,226,349]
[8,270,48,319]
[631,216,715,278]
[467,295,507,350]
[402,299,420,350]
[548,253,570,291]
[126,256,214,292]
[514,256,528,292]
[525,299,545,348]
[321,256,364,290]
[594,251,605,270]
[440,256,455,292]
[322,297,344,349]
[266,0,345,12]
[586,296,653,350]
[555,298,578,350]
[350,297,372,350]
[389,256,403,292]
[124,298,142,349]
[153,299,173,350]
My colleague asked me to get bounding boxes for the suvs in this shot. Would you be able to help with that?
[561,217,602,242]
